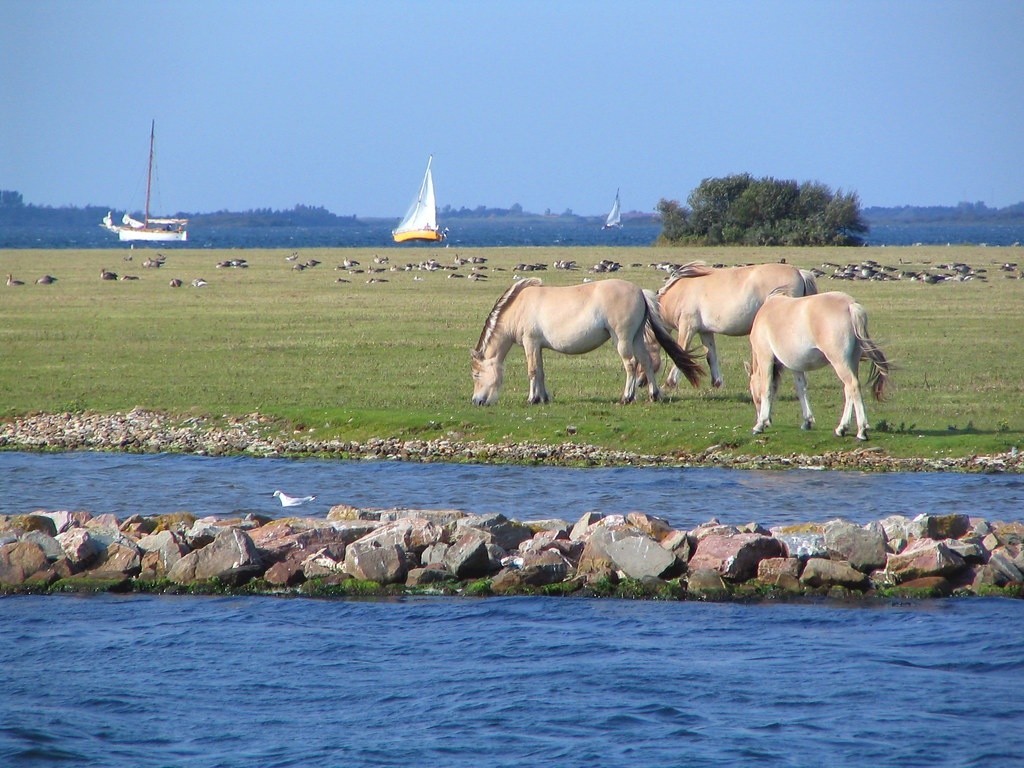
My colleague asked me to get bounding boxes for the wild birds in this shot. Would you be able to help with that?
[272,490,316,507]
[34,275,56,285]
[6,274,24,286]
[285,252,1024,284]
[100,252,248,287]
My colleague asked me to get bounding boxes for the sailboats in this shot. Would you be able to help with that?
[603,187,623,231]
[98,118,188,241]
[392,153,450,244]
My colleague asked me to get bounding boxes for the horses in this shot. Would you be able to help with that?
[470,277,709,407]
[633,259,817,398]
[742,284,900,443]
